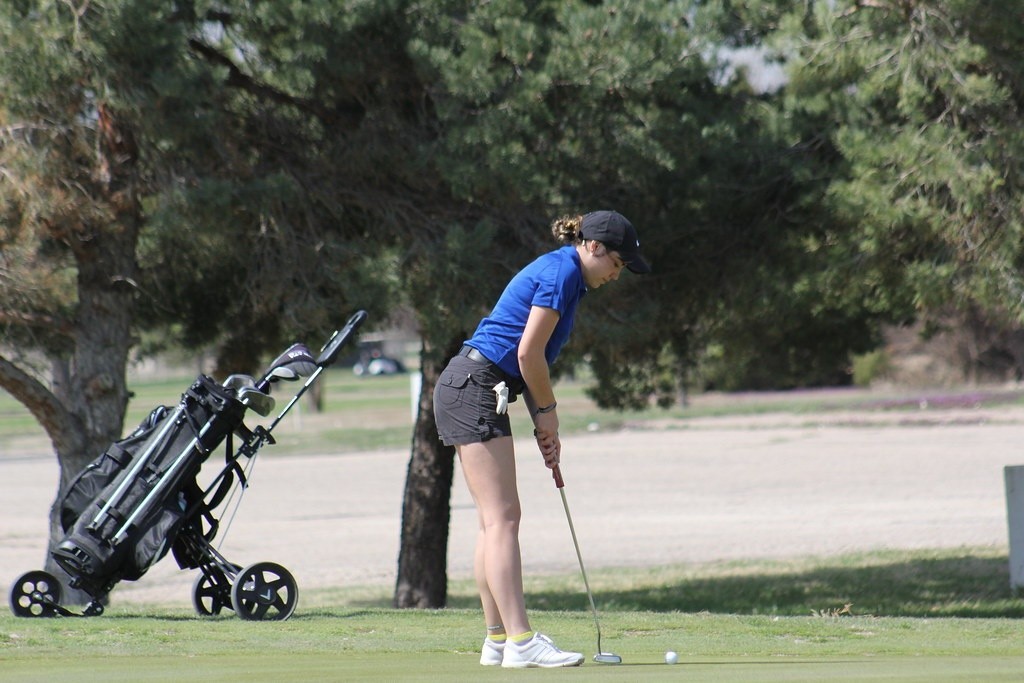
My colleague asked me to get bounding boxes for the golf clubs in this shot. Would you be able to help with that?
[539,433,622,665]
[223,342,319,417]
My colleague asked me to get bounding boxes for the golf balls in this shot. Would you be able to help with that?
[664,651,678,665]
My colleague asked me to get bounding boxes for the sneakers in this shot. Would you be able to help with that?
[483,634,584,669]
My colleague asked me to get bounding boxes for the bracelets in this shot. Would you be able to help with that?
[536,402,557,414]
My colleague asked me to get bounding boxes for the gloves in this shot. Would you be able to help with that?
[494,381,508,415]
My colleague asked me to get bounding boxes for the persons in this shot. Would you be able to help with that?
[433,210,649,667]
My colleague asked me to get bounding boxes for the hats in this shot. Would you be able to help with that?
[580,208,650,278]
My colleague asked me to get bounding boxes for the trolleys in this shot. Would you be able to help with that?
[12,310,366,618]
